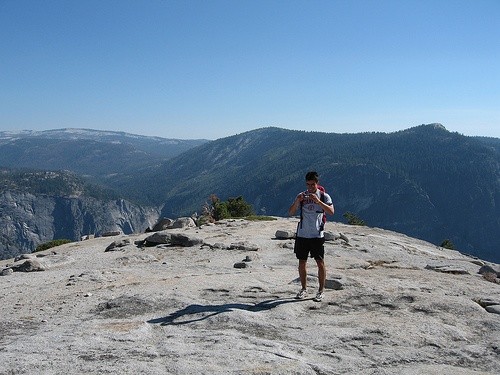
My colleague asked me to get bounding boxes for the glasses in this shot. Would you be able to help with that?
[305,181,316,187]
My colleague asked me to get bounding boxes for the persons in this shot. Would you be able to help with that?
[288,171,335,302]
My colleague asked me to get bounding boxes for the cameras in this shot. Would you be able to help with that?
[304,193,312,198]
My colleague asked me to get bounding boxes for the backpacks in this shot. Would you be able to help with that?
[300,184,327,235]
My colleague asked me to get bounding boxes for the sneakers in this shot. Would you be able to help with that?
[296,289,308,298]
[315,289,325,301]
